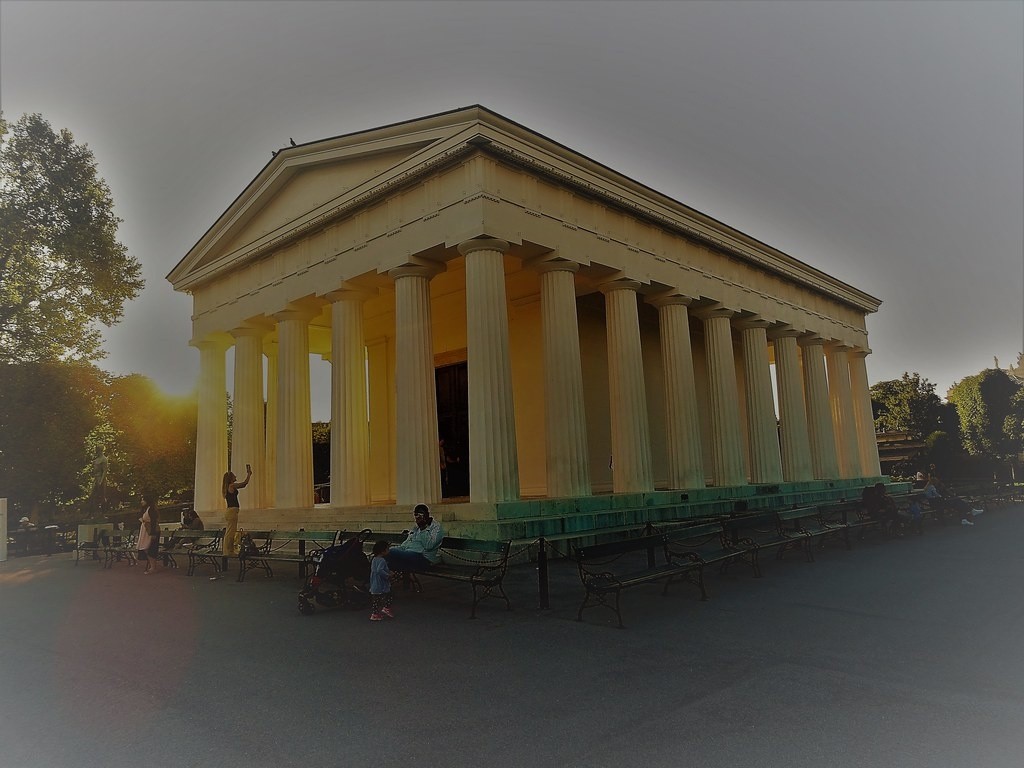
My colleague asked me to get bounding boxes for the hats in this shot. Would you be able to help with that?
[18,516,30,523]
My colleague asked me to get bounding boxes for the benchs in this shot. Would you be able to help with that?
[72,529,511,620]
[571,479,1024,630]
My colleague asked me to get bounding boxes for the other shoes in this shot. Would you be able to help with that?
[370,614,383,620]
[382,608,395,619]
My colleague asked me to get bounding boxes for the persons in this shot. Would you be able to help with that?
[222,465,253,558]
[868,479,985,539]
[380,502,449,570]
[163,508,206,550]
[368,540,392,620]
[137,495,162,575]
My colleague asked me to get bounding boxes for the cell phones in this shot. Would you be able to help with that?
[418,513,424,519]
[246,465,250,472]
